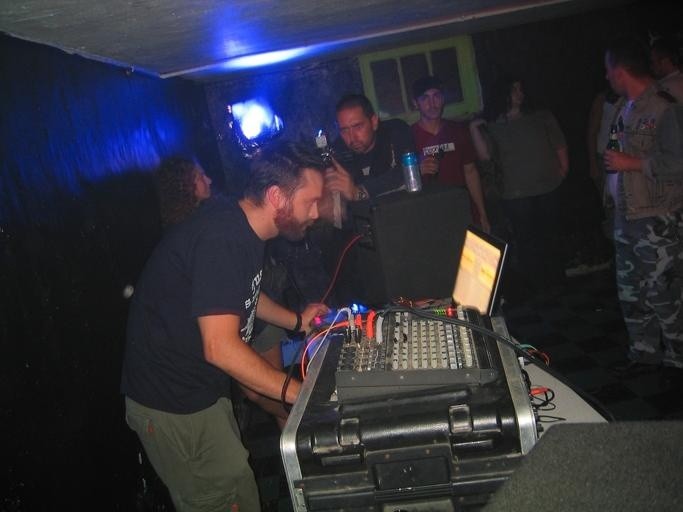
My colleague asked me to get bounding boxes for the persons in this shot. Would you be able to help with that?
[467,68,571,297]
[119,139,333,511]
[649,38,682,112]
[410,75,491,236]
[325,92,414,203]
[588,74,621,199]
[600,35,682,386]
[150,156,213,236]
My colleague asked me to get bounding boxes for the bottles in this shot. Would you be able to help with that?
[604,125,621,174]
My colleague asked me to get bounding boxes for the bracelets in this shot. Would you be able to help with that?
[357,185,367,201]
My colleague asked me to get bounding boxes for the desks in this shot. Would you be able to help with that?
[516,353,614,443]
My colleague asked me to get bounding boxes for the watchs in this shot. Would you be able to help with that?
[284,311,302,336]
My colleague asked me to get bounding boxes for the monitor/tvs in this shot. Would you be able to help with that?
[451,227,509,316]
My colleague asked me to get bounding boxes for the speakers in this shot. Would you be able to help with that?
[346,186,475,307]
